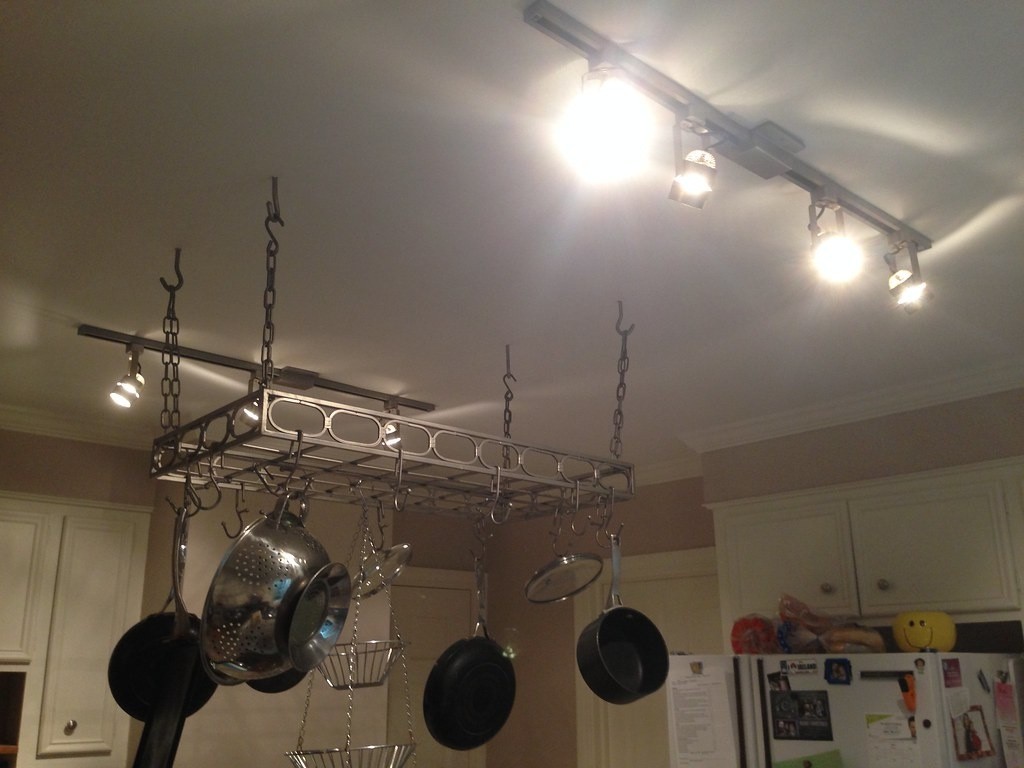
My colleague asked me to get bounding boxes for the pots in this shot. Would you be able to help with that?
[577,535,670,705]
[105,508,218,720]
[423,555,515,751]
[246,667,308,693]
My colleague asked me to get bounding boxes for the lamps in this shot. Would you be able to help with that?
[883,244,930,300]
[803,197,863,281]
[669,118,716,213]
[107,343,151,408]
[378,403,407,446]
[238,368,270,425]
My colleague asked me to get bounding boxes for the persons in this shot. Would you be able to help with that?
[831,663,846,679]
[908,716,916,737]
[964,713,982,752]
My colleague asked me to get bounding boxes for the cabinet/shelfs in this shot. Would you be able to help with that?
[703,456,1024,628]
[0,493,157,768]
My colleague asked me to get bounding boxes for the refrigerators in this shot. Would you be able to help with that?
[736,647,1024,768]
